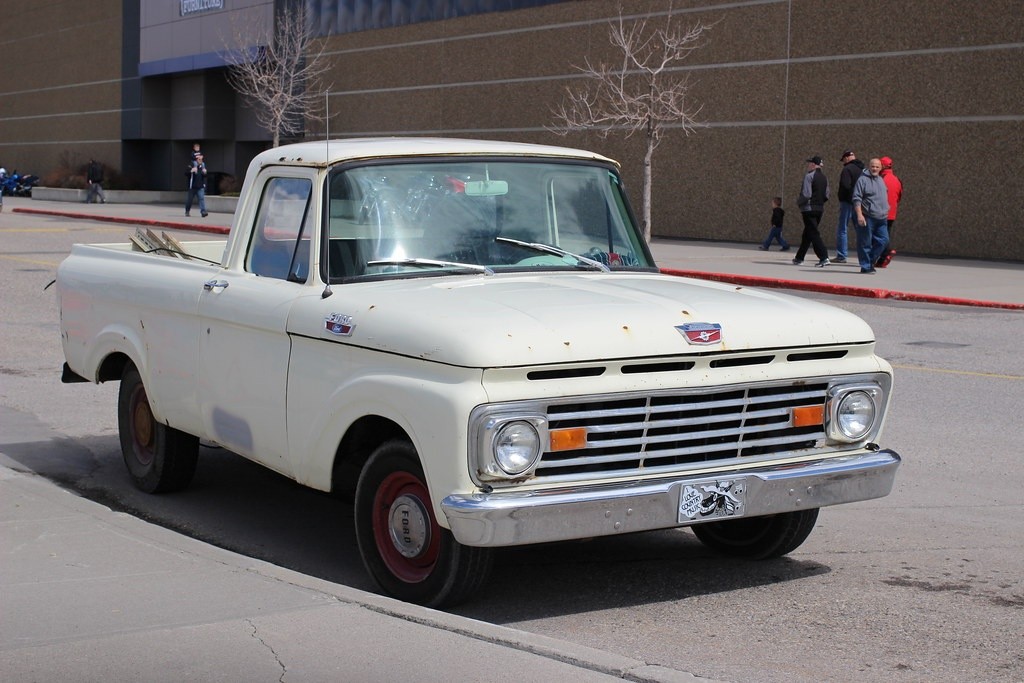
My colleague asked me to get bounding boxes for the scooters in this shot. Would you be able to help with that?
[0,169,39,197]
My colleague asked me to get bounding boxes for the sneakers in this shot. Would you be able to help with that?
[792,258,804,265]
[815,257,830,268]
[831,256,847,263]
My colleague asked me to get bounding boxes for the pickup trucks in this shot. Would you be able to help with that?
[53,135,902,620]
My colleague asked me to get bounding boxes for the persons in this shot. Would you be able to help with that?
[852,158,889,273]
[190,143,206,174]
[875,156,902,267]
[82,157,107,204]
[0,168,19,207]
[758,197,790,252]
[276,180,308,200]
[792,157,831,267]
[829,151,869,263]
[184,156,208,217]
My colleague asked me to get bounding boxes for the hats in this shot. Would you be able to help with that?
[806,155,823,167]
[881,157,892,168]
[840,150,854,161]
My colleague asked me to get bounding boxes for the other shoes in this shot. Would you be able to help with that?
[864,266,877,274]
[875,250,896,268]
[780,245,789,251]
[756,244,768,251]
